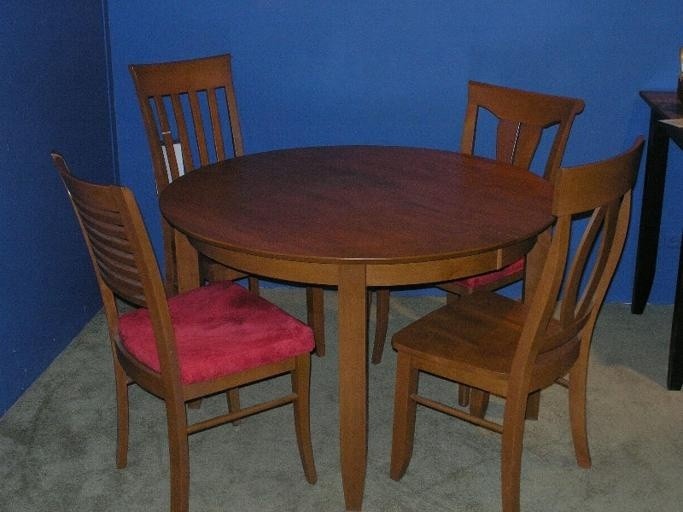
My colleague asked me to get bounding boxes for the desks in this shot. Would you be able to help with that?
[631,91,683,390]
